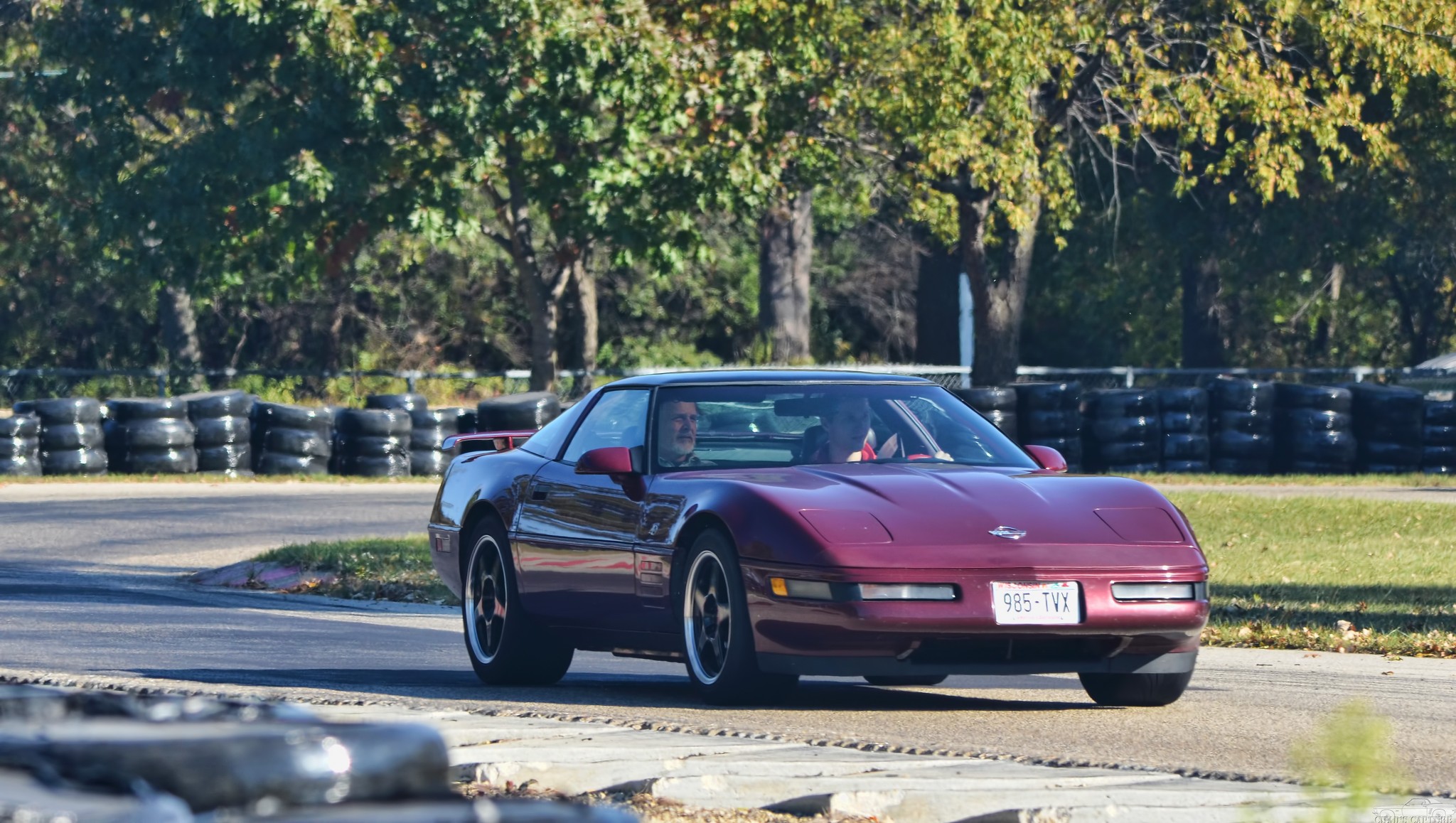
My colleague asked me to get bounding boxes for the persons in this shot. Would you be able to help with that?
[812,396,954,465]
[657,399,719,466]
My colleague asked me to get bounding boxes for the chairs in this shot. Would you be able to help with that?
[800,425,877,464]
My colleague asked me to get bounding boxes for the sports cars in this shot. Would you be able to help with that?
[426,368,1214,708]
